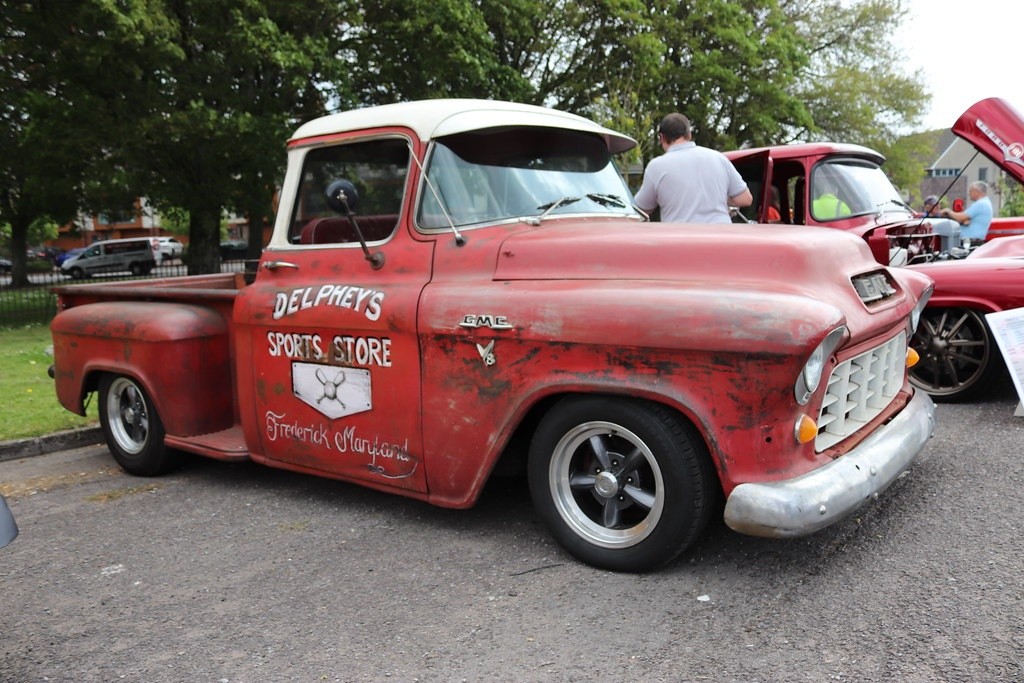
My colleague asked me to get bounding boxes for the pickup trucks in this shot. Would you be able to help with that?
[46,96,939,575]
[720,97,1024,406]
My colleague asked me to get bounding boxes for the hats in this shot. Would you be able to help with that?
[924,196,943,206]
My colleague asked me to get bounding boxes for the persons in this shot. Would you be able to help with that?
[914,196,944,218]
[758,186,781,223]
[813,182,852,220]
[940,180,994,246]
[632,113,753,224]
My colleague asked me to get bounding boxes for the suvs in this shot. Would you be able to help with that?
[156,236,185,261]
[61,236,163,280]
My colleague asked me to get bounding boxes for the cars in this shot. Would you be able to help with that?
[0,245,96,278]
[218,238,249,263]
